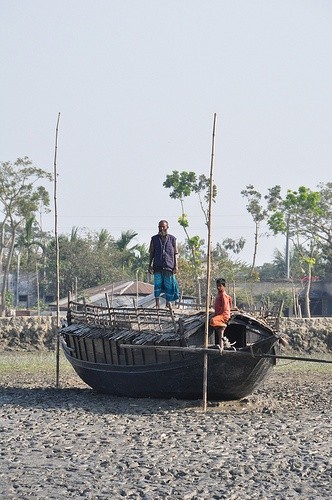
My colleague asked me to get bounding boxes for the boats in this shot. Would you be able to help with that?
[60,291,281,401]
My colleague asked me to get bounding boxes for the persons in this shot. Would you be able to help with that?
[148,220,179,310]
[208,278,233,350]
